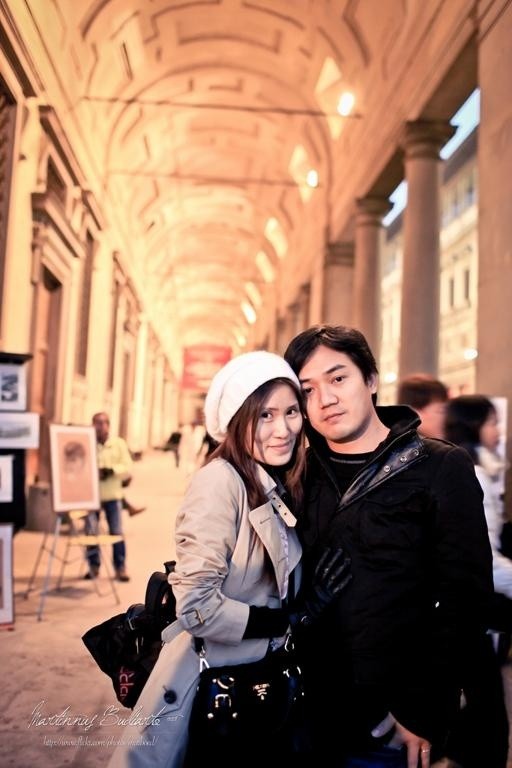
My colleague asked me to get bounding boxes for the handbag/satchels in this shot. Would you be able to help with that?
[123,482,147,515]
[187,654,314,766]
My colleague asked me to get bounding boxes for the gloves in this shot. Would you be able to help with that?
[290,546,353,635]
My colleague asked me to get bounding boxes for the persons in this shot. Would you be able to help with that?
[84,412,146,581]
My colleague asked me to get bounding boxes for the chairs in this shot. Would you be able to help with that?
[56,510,124,602]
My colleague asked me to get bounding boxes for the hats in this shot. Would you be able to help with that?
[205,350,301,443]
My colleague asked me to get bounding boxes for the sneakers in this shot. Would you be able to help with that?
[84,567,99,578]
[116,570,129,581]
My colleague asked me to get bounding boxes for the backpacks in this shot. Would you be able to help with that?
[81,572,176,708]
